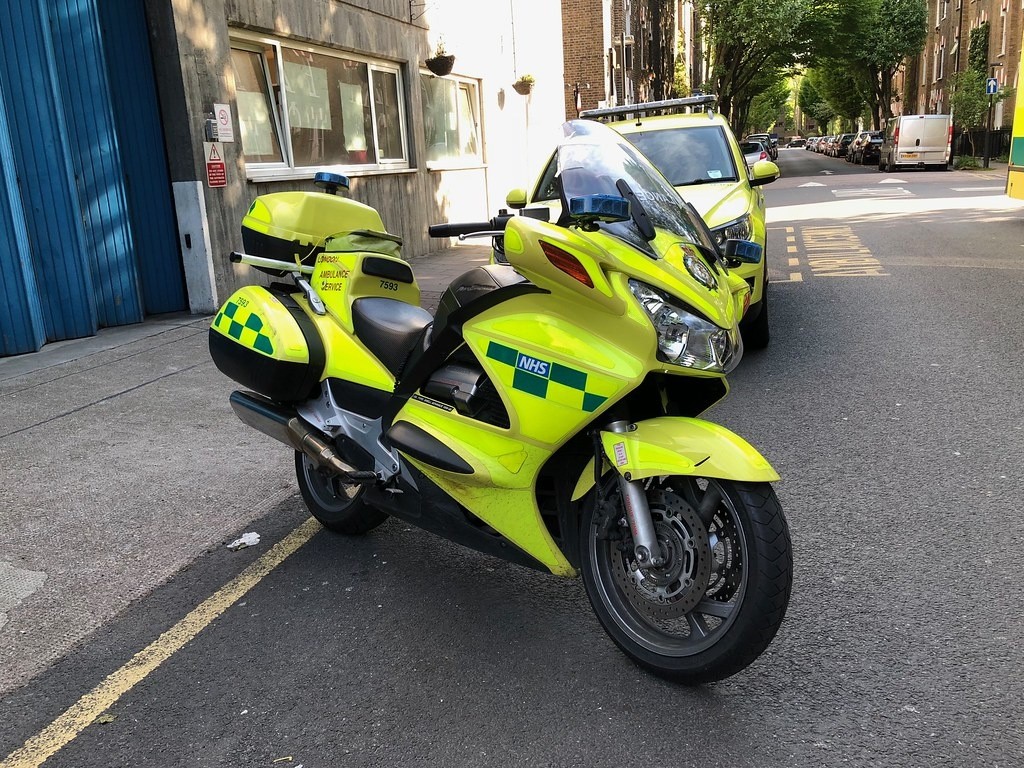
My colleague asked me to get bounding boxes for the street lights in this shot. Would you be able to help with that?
[983,63,1003,168]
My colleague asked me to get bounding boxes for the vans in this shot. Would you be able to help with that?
[792,139,807,146]
[879,114,953,172]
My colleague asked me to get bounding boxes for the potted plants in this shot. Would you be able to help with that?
[423,32,454,77]
[512,75,536,95]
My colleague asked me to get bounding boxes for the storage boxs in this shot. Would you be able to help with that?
[208,286,325,403]
[241,190,385,278]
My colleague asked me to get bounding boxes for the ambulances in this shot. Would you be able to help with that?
[208,119,793,685]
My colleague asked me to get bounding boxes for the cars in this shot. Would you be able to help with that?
[835,133,856,158]
[805,135,840,157]
[786,140,804,148]
[738,140,771,169]
[854,134,883,165]
[746,134,778,160]
[506,94,780,345]
[846,131,879,162]
